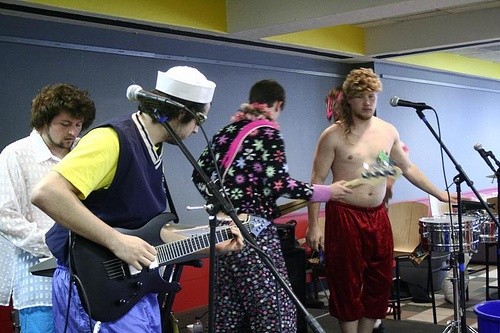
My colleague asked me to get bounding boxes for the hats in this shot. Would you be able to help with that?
[156,66,216,103]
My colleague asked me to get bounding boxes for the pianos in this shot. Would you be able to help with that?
[26,222,210,333]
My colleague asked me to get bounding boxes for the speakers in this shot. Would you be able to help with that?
[280,247,308,333]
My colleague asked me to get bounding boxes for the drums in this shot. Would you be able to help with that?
[443,208,500,245]
[417,214,480,254]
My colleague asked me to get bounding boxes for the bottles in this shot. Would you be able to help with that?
[193,316,203,333]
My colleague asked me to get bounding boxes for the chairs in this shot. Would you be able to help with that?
[388,201,437,325]
[484,197,500,302]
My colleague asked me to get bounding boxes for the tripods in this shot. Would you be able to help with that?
[442,255,478,333]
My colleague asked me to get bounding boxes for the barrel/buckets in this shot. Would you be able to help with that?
[473,300,500,333]
[396,252,450,297]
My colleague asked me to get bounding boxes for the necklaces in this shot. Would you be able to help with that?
[40,133,54,154]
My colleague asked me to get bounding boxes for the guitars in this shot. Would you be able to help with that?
[67,214,273,324]
[273,159,404,220]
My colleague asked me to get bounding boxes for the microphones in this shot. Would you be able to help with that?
[389,96,433,110]
[126,85,185,110]
[474,143,496,172]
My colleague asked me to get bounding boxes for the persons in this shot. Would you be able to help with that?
[305,67,471,333]
[31,65,245,333]
[0,82,97,333]
[191,79,353,333]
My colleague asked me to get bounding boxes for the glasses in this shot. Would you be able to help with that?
[196,112,207,125]
